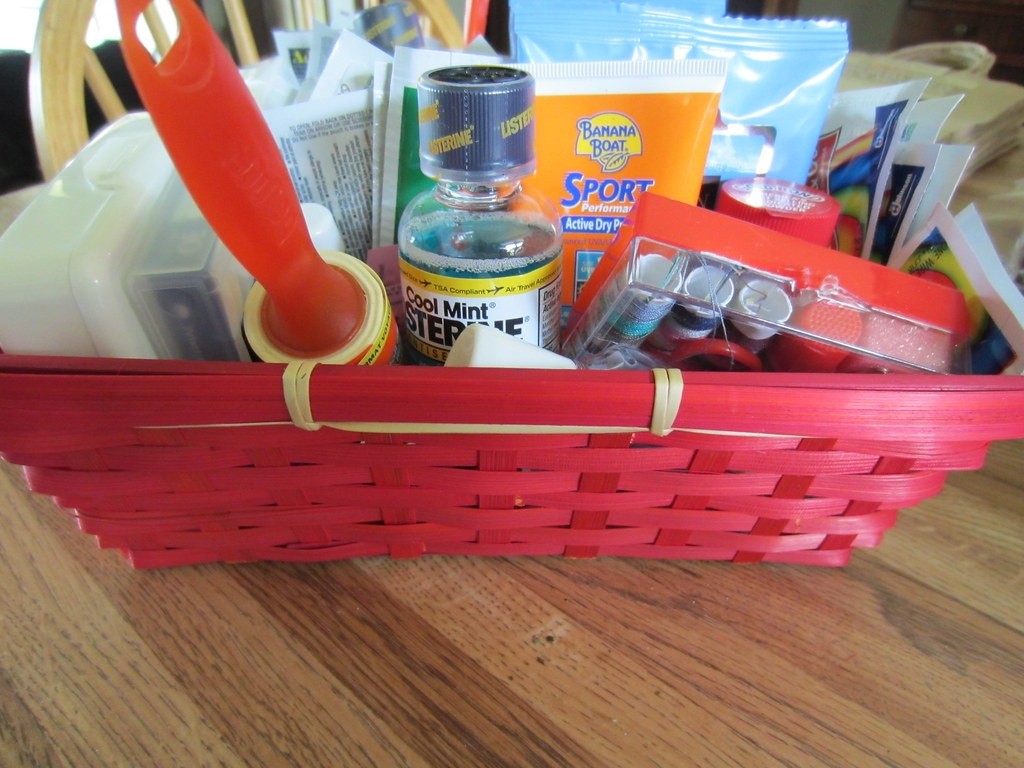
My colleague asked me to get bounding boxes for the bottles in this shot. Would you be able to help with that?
[397,63,563,369]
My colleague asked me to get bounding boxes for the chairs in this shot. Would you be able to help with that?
[34,0,262,187]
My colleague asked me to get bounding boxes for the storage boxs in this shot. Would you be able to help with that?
[0,106,160,361]
[123,157,258,363]
[562,190,973,375]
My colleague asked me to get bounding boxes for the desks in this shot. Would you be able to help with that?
[0,143,1024,768]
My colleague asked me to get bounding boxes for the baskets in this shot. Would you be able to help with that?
[1,348,1024,570]
